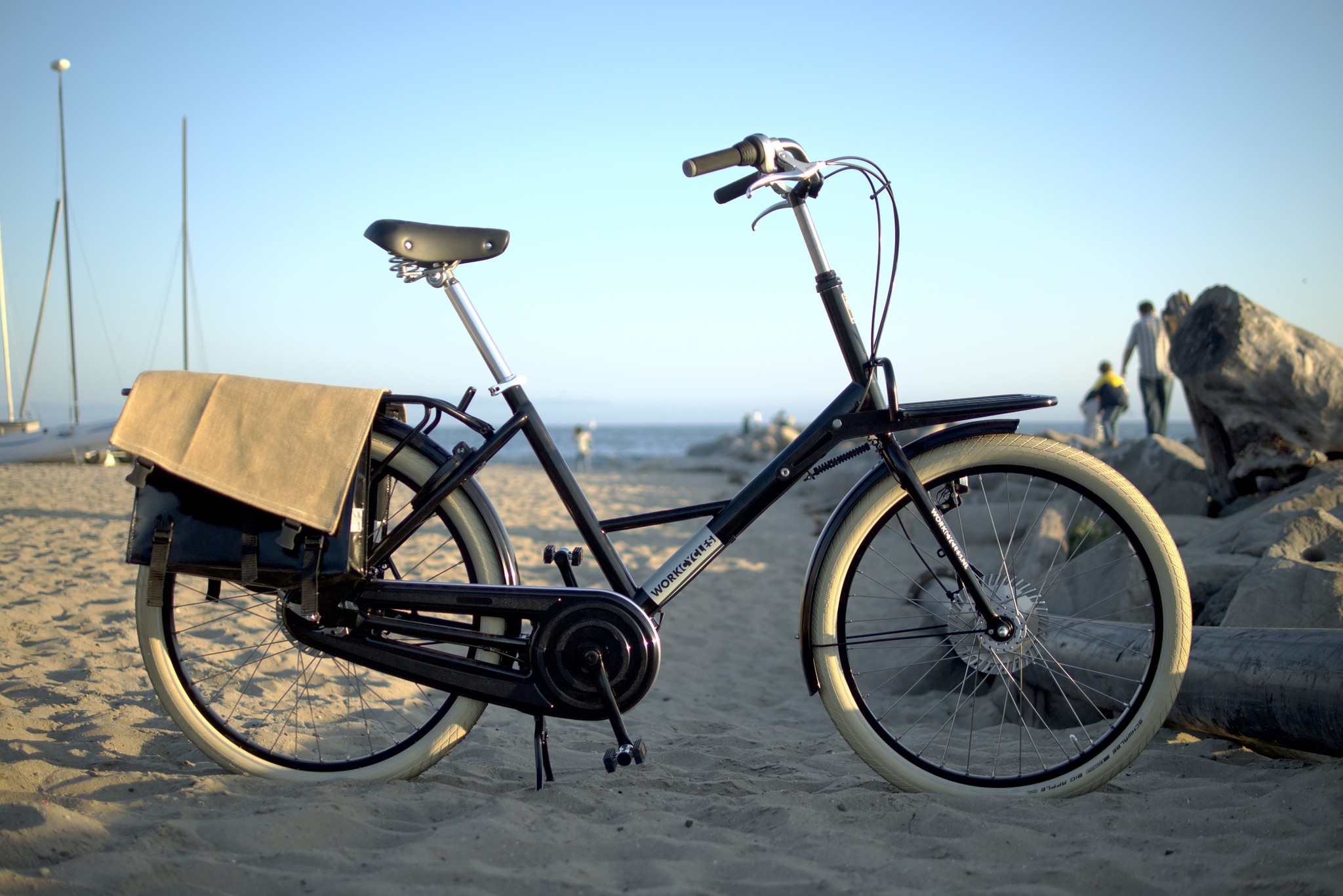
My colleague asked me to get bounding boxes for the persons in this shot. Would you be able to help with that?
[1119,301,1174,438]
[572,426,594,475]
[1082,362,1128,450]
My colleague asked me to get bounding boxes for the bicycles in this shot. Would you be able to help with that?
[121,134,1192,802]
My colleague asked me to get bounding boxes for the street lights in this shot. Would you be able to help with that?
[52,60,79,427]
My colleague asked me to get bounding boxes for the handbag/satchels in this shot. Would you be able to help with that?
[112,369,405,614]
